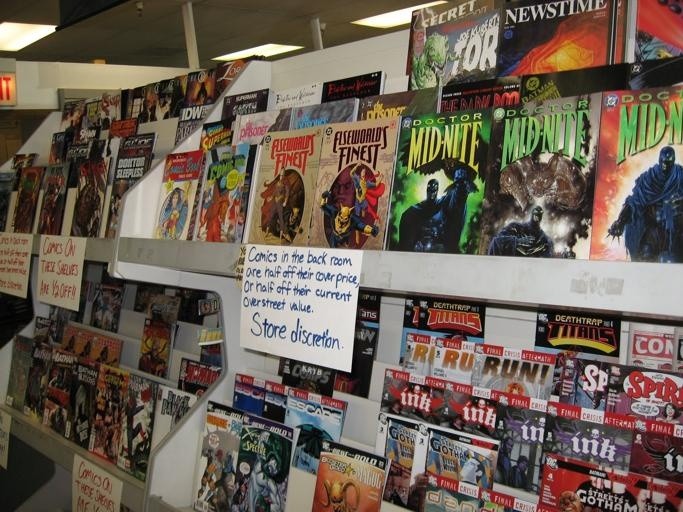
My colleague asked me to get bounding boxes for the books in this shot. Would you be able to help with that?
[1,0,682,510]
